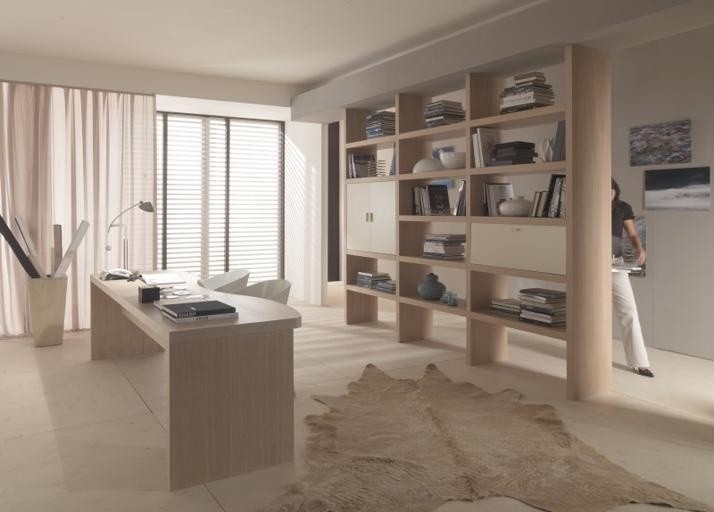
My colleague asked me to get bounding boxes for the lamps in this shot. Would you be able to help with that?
[106,201,153,272]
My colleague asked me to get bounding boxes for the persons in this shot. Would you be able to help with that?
[611,177,655,378]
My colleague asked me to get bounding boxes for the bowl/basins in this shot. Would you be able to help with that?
[411,150,466,174]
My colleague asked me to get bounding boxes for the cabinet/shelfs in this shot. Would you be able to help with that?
[343,44,566,393]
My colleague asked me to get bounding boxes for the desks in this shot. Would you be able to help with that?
[87,272,299,491]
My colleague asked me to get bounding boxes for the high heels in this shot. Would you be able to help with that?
[633,367,654,377]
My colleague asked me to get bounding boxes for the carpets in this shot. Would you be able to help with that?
[257,360,714,509]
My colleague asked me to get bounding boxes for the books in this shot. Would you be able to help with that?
[349,150,372,177]
[138,273,186,285]
[365,110,396,137]
[498,72,555,113]
[152,296,207,311]
[472,119,565,168]
[411,179,466,216]
[161,311,239,323]
[162,300,236,319]
[491,288,568,328]
[356,271,397,294]
[420,233,467,260]
[482,173,567,218]
[424,100,467,127]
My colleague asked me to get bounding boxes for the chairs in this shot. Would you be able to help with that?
[195,268,289,305]
